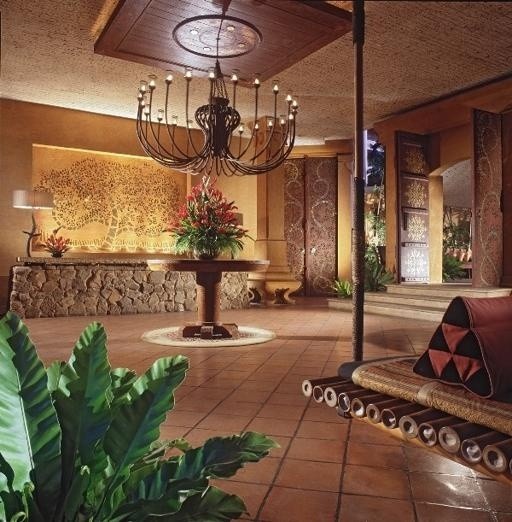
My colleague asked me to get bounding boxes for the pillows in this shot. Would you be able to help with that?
[412,295,512,397]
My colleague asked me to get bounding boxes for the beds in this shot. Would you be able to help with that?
[301,358,512,483]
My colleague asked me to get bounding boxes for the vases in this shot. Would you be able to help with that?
[193,241,222,262]
[52,252,62,258]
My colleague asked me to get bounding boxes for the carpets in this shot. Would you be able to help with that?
[143,321,274,346]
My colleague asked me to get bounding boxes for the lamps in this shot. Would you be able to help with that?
[135,1,299,177]
[13,190,54,256]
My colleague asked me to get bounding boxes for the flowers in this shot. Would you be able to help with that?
[159,177,257,261]
[44,234,70,255]
